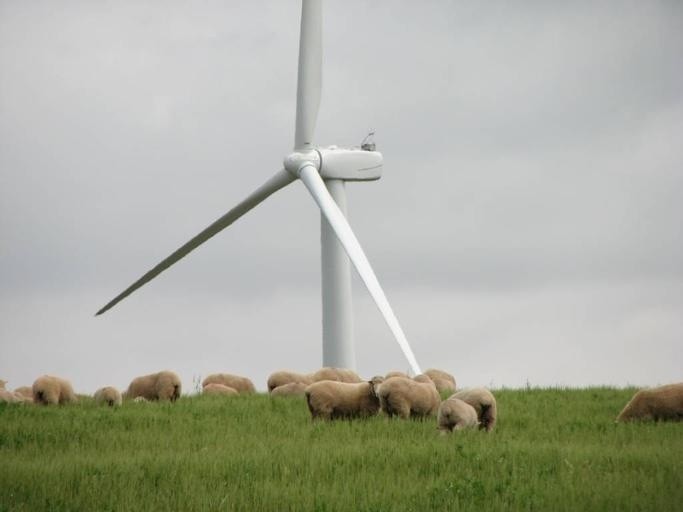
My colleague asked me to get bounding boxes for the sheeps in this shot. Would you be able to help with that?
[93,386,123,406]
[0,378,32,404]
[266,366,498,436]
[32,373,77,405]
[128,370,183,400]
[613,381,683,424]
[199,372,256,399]
